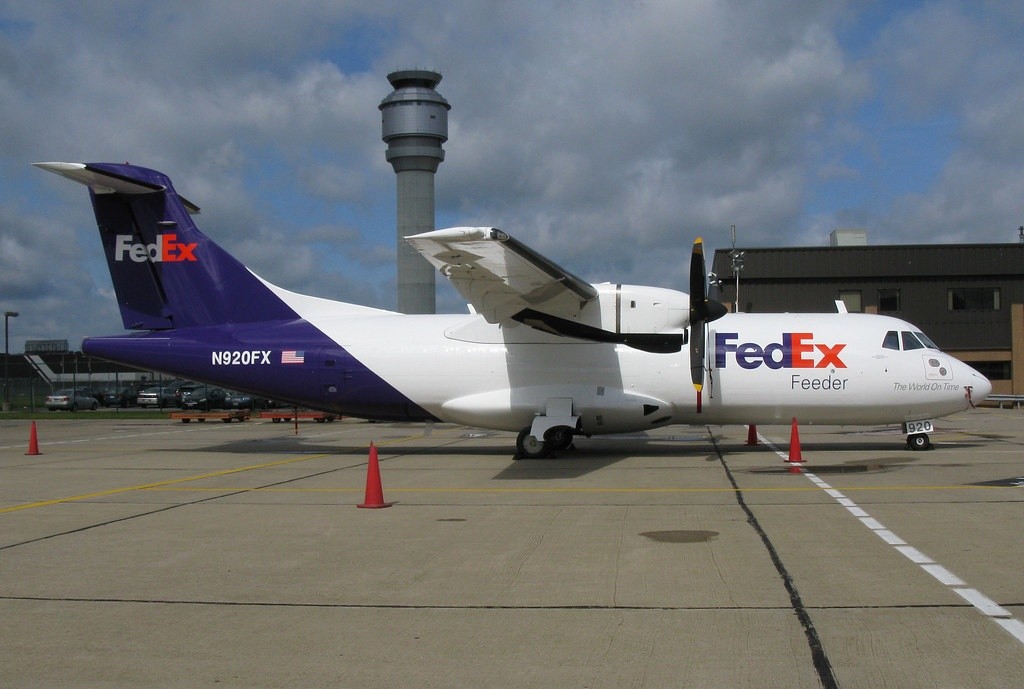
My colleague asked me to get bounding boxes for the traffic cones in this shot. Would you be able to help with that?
[357,440,392,508]
[24,421,42,455]
[745,424,761,446]
[783,416,807,462]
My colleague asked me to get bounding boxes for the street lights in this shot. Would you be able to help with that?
[2,312,19,411]
[730,249,746,313]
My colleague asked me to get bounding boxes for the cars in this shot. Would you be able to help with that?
[44,389,99,411]
[228,391,255,409]
[252,395,293,410]
[137,387,180,408]
[101,385,140,407]
[180,388,232,411]
[72,386,103,407]
[170,381,206,408]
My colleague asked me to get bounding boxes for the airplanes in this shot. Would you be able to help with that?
[30,160,993,459]
